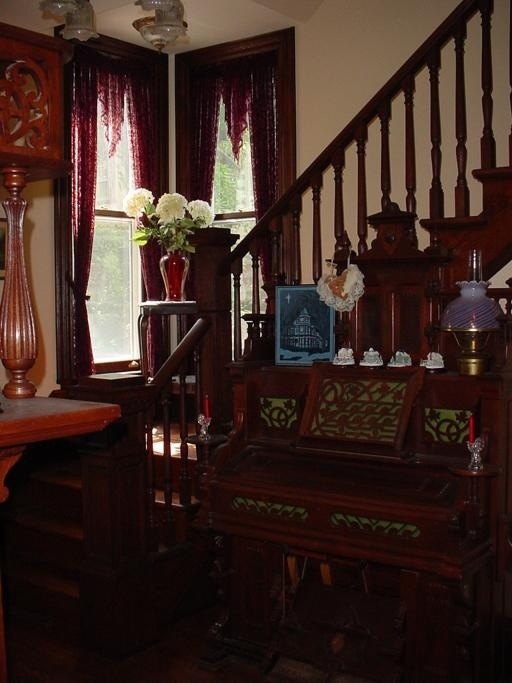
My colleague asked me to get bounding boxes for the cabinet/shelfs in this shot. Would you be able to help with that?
[0,21,73,181]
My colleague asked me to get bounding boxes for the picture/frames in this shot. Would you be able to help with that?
[274,284,336,366]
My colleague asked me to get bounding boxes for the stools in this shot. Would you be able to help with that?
[137,301,198,428]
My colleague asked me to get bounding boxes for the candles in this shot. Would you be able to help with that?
[469,413,475,447]
[204,394,209,423]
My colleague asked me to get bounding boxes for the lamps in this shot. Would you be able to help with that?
[132,16,188,54]
[442,281,506,377]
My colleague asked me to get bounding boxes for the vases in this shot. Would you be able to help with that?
[159,247,189,301]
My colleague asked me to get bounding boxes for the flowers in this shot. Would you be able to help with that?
[124,187,216,253]
